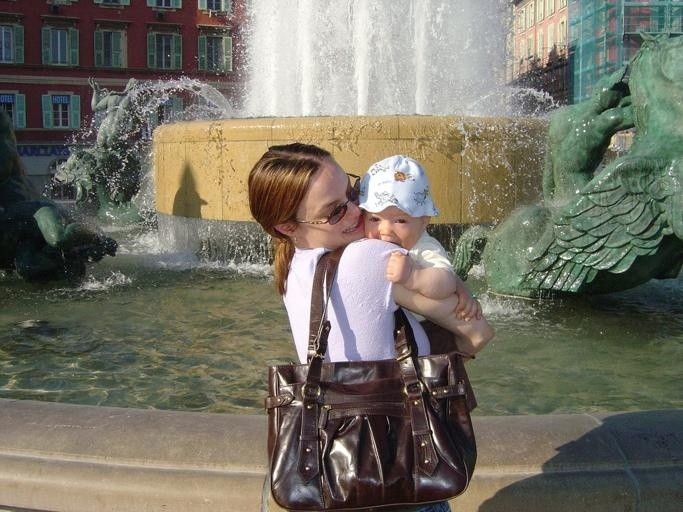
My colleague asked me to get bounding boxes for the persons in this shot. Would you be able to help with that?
[357,150,461,310]
[246,143,497,510]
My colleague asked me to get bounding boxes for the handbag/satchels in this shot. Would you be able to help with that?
[263,346,481,512]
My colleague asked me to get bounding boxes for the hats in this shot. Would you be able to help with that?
[356,153,440,221]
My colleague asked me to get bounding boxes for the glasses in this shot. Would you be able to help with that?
[290,169,362,227]
